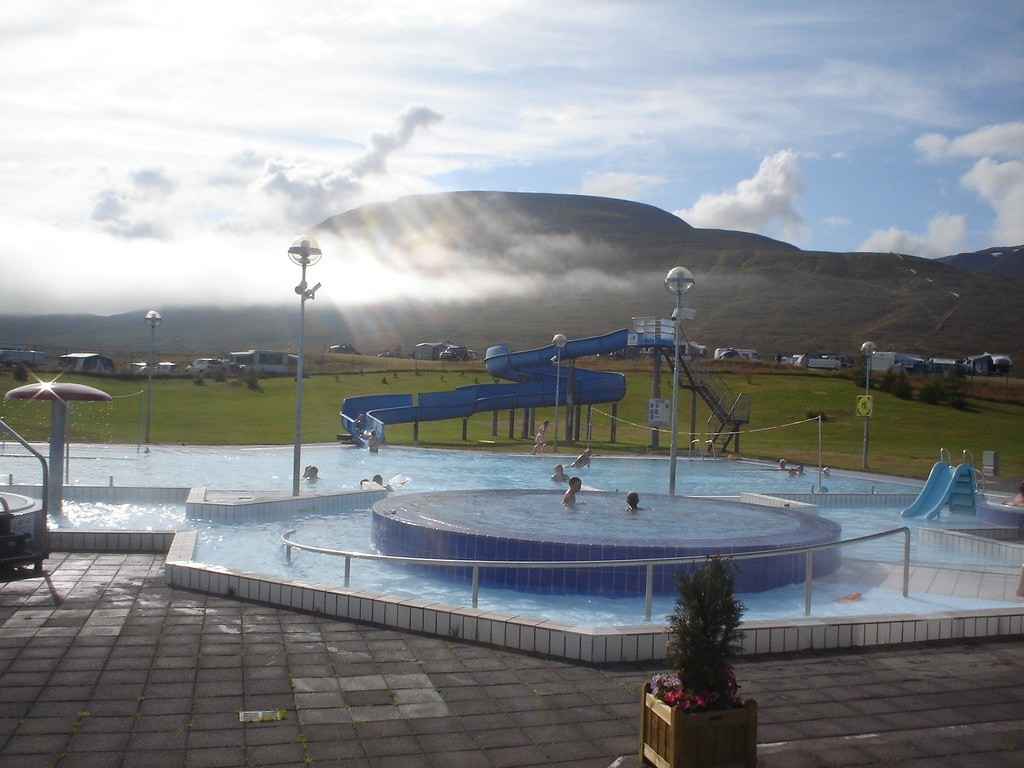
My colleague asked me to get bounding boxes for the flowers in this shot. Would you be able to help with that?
[650,667,745,709]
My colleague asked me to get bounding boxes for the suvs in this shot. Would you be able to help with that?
[159,361,179,377]
[184,358,223,377]
[440,344,469,363]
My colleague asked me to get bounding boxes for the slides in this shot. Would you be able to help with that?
[338,326,673,448]
[899,460,972,521]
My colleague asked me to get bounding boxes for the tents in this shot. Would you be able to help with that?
[414,342,444,361]
[59,351,115,374]
[718,349,740,361]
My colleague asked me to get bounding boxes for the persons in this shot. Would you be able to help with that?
[355,414,364,437]
[368,429,378,452]
[562,447,592,469]
[623,492,643,512]
[1010,480,1024,507]
[560,477,582,505]
[779,459,786,469]
[777,353,781,364]
[820,467,830,478]
[361,475,394,492]
[531,420,550,455]
[1015,564,1024,595]
[788,464,803,477]
[832,590,863,603]
[303,465,322,482]
[550,464,569,482]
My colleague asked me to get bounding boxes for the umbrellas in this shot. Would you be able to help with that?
[5,381,113,484]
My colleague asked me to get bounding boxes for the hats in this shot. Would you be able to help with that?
[823,467,829,474]
[626,492,639,503]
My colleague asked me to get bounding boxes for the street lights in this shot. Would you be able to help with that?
[286,235,323,498]
[554,332,567,451]
[665,266,696,495]
[146,310,162,440]
[861,341,877,468]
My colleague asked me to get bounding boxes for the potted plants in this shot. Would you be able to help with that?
[639,553,757,768]
